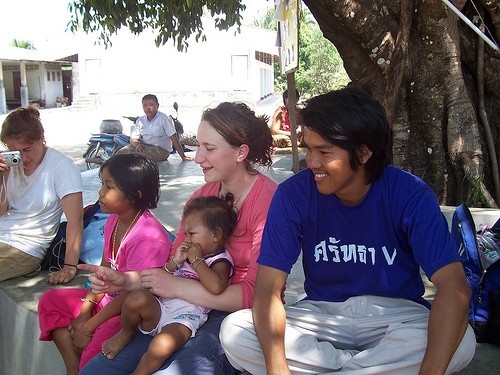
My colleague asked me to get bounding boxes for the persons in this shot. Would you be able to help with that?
[0,105,83,284]
[37,154,171,375]
[269,89,305,148]
[101,192,239,375]
[219,87,477,375]
[76,102,287,375]
[115,95,194,162]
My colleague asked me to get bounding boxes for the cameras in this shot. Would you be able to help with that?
[0,151,22,165]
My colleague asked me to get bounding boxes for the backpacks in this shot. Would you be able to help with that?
[451,202,500,343]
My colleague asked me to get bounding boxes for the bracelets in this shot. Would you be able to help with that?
[164,262,175,274]
[191,258,205,269]
[170,257,177,267]
[64,263,77,269]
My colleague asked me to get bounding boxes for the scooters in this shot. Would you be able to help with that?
[82,101,184,172]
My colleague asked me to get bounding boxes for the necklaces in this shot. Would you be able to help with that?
[111,210,143,268]
[233,176,257,204]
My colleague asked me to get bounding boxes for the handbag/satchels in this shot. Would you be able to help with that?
[40,200,100,271]
